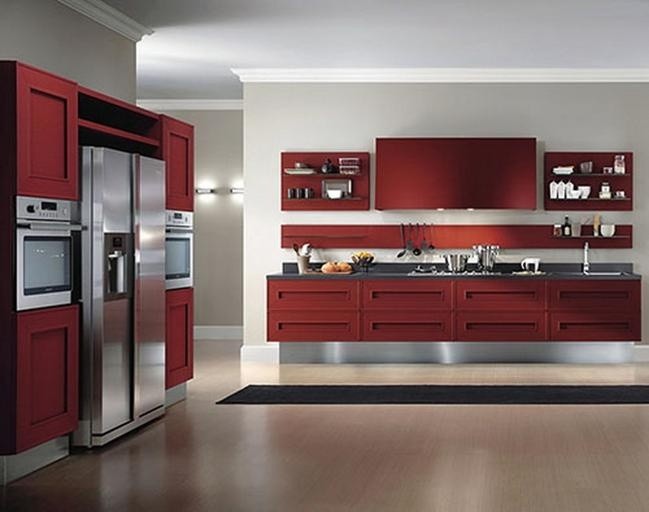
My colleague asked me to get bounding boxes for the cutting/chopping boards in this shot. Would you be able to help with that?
[305,271,354,275]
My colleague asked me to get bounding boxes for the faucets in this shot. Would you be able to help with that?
[583,242,590,273]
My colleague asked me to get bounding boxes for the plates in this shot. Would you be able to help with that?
[553,168,573,175]
[284,167,315,174]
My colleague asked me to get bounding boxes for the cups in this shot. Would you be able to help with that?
[296,255,309,274]
[286,187,312,200]
[602,166,612,174]
[571,223,582,237]
[616,191,625,198]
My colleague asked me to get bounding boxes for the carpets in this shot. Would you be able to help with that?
[215,385,648,403]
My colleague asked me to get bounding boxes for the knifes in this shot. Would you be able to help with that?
[355,256,374,266]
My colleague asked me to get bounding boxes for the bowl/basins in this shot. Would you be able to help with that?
[597,192,612,199]
[600,225,615,237]
[570,185,591,199]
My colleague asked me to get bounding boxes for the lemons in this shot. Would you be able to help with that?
[354,251,372,258]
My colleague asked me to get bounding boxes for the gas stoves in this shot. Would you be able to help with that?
[413,266,497,274]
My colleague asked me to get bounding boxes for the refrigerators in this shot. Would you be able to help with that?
[78,144,165,448]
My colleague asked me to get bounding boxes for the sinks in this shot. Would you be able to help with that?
[547,272,630,280]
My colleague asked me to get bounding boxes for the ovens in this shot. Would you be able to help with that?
[16,195,83,312]
[165,209,195,291]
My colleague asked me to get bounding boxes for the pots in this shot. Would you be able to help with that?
[446,254,468,271]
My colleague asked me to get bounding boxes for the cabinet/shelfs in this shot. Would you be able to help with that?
[543,150,634,213]
[79,85,161,149]
[4,304,81,454]
[160,114,195,211]
[1,60,80,201]
[165,288,194,389]
[264,276,643,343]
[280,152,371,213]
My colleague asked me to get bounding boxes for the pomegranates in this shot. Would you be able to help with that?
[322,261,353,274]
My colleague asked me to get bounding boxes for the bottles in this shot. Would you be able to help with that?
[601,182,610,192]
[561,215,572,236]
[614,155,625,174]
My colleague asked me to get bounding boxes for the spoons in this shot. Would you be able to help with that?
[293,244,300,255]
